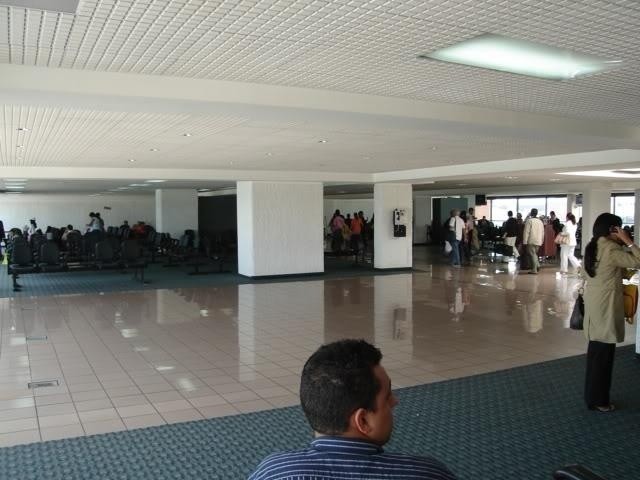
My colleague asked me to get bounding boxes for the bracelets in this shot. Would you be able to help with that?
[627,242,634,248]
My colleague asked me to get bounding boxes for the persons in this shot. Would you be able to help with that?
[328,208,369,251]
[581,212,640,412]
[0,212,145,264]
[250,339,462,480]
[441,208,582,274]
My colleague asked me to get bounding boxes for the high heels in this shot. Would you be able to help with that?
[586,401,616,412]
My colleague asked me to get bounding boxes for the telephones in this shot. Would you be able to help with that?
[393,208,408,225]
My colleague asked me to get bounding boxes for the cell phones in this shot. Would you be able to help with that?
[609,225,618,233]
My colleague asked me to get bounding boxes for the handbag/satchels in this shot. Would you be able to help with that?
[331,220,343,232]
[570,295,584,330]
[554,232,569,244]
[497,243,513,256]
[440,241,452,256]
[445,230,456,241]
[623,284,638,318]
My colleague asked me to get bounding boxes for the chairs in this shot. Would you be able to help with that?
[476,221,507,252]
[7,226,225,292]
